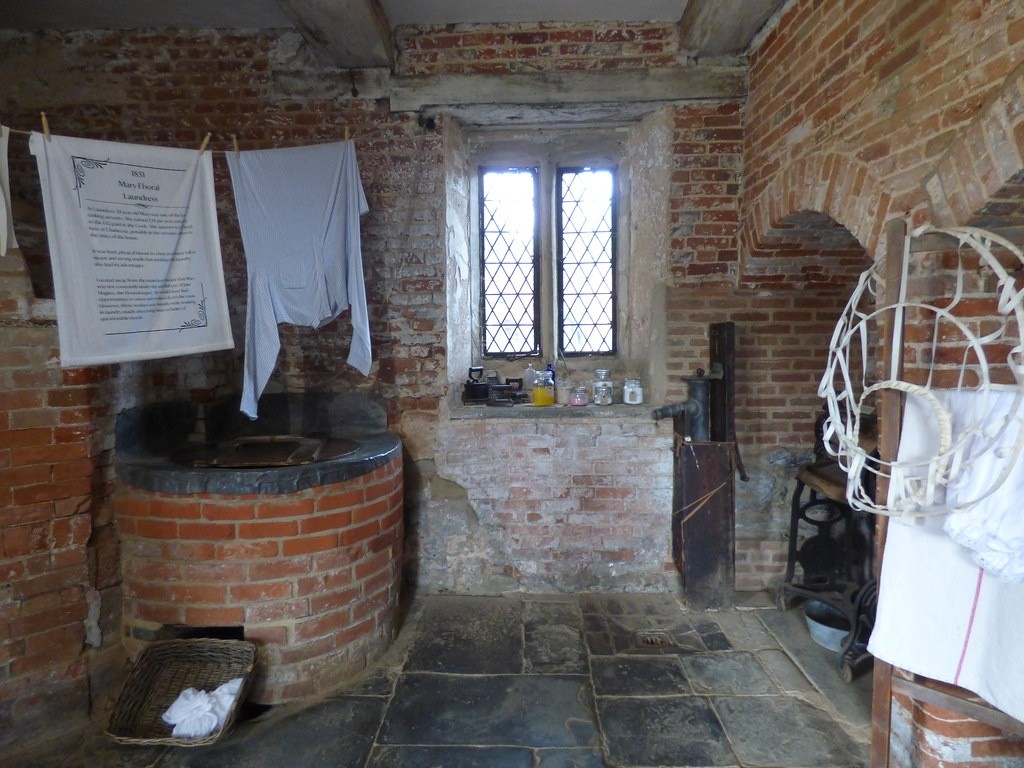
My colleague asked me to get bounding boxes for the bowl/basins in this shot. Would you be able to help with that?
[802,599,851,652]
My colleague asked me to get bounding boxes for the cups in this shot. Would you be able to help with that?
[555,387,571,404]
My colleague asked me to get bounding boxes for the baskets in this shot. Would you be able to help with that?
[104,637,256,747]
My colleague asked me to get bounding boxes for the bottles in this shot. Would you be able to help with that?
[571,387,588,406]
[523,364,535,403]
[600,389,608,405]
[592,370,614,404]
[624,377,643,405]
[534,371,554,406]
[544,364,555,386]
